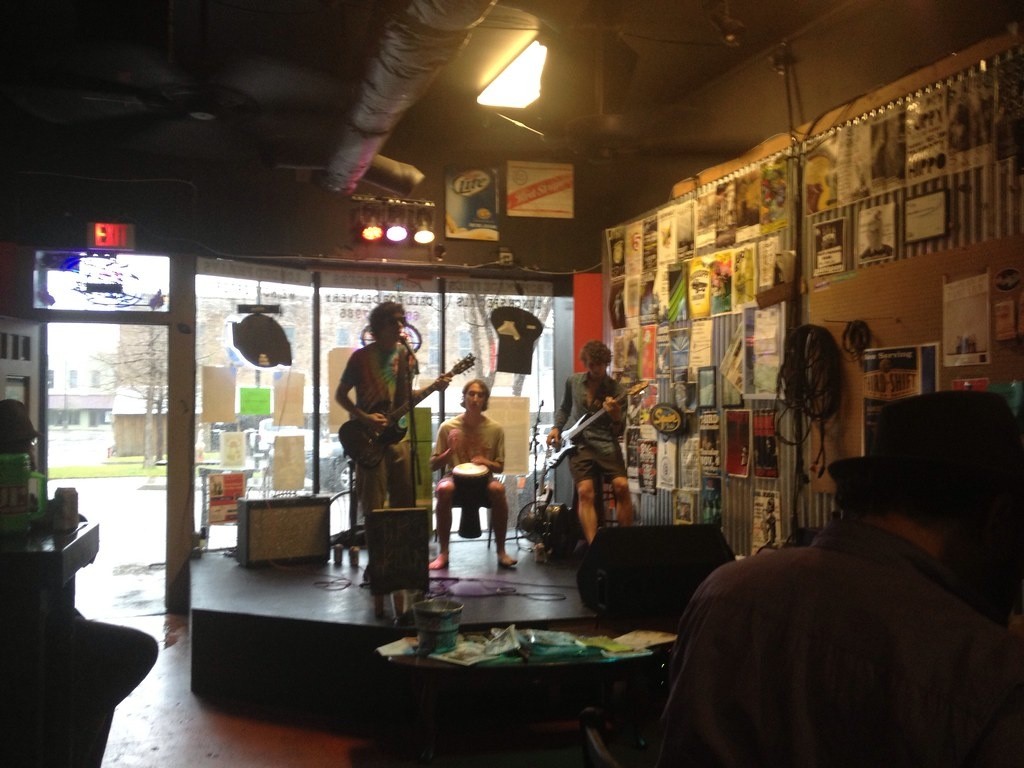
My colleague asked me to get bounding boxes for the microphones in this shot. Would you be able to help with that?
[396,333,417,361]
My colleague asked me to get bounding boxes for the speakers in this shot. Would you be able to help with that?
[366,507,432,595]
[236,497,332,566]
[578,522,735,622]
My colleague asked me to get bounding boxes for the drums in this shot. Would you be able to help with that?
[452,462,490,539]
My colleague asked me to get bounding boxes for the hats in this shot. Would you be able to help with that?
[827,389,1024,514]
[0,399,42,444]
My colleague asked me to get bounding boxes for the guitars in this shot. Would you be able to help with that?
[337,351,477,471]
[545,379,651,469]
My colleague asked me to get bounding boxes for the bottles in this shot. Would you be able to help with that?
[0,451,48,537]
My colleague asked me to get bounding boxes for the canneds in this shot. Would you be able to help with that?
[536,543,544,563]
[349,546,359,567]
[53,487,79,534]
[334,544,344,564]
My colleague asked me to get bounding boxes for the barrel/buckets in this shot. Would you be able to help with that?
[390,590,424,622]
[412,599,464,654]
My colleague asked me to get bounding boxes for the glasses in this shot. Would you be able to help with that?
[587,360,604,368]
[383,315,407,326]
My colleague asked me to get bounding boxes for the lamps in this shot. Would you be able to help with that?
[348,194,439,244]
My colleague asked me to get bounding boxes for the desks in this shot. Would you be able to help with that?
[0,522,99,767]
[388,631,648,768]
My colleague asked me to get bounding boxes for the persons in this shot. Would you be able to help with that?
[648,391,1024,768]
[0,399,157,768]
[683,505,691,522]
[547,340,635,547]
[428,380,517,570]
[336,302,451,582]
[860,220,893,259]
